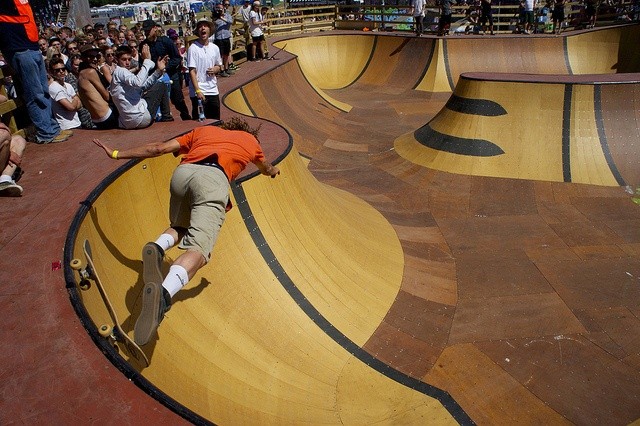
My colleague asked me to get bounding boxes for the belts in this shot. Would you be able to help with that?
[193,161,226,174]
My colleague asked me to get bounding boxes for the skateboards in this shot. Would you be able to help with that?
[69,239,150,368]
[268,44,287,60]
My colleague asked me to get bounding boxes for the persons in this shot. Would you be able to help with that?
[481,1,495,35]
[49,35,72,71]
[234,0,271,62]
[44,27,54,39]
[48,58,83,130]
[0,122,25,196]
[0,0,73,143]
[79,20,192,129]
[37,37,48,58]
[212,1,240,78]
[47,46,61,59]
[132,6,196,23]
[412,0,426,37]
[188,18,224,121]
[269,11,302,25]
[65,42,80,71]
[509,0,637,34]
[437,1,452,36]
[71,55,82,77]
[58,26,75,43]
[92,115,281,346]
[311,10,362,23]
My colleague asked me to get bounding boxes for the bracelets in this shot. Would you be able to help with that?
[111,150,119,158]
[195,89,202,94]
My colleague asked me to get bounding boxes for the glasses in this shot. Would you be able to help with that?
[50,66,68,73]
[107,52,114,56]
[67,46,77,49]
[223,3,230,5]
[83,53,103,60]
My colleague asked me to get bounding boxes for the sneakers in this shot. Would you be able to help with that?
[142,241,164,286]
[0,179,24,198]
[58,129,73,138]
[53,135,66,142]
[134,282,166,345]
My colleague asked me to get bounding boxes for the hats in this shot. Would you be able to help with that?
[193,15,216,37]
[94,21,104,28]
[78,44,103,59]
[49,35,60,41]
[142,19,156,31]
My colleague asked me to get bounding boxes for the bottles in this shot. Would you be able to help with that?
[197,99,206,122]
